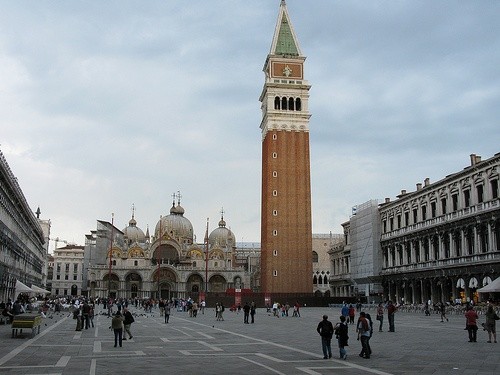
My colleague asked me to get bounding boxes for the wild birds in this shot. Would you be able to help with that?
[131,310,155,318]
[212,325,215,328]
[213,309,214,311]
[60,313,65,317]
[108,326,112,329]
[94,310,108,316]
[44,313,59,327]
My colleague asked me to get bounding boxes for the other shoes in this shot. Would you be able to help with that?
[122,338,127,340]
[323,357,327,359]
[474,340,477,342]
[329,353,332,358]
[363,356,370,359]
[359,354,363,357]
[494,341,497,343]
[129,336,133,339]
[344,355,347,359]
[487,341,491,343]
[468,340,473,342]
[120,342,122,346]
[114,345,117,347]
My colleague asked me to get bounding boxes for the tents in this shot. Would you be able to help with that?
[477,277,500,302]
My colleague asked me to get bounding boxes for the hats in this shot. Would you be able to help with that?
[116,311,121,315]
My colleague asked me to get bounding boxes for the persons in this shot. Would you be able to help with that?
[243,300,256,324]
[465,305,478,343]
[216,303,225,321]
[485,305,500,343]
[73,299,94,331]
[342,303,355,324]
[425,298,448,322]
[267,300,300,318]
[386,301,395,332]
[317,315,334,359]
[356,302,362,311]
[0,292,113,324]
[122,308,135,340]
[356,312,373,359]
[230,303,242,315]
[377,302,385,332]
[115,297,198,323]
[464,302,471,330]
[200,300,205,314]
[333,316,349,360]
[112,311,125,347]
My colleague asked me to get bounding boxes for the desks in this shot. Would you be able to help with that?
[11,325,40,337]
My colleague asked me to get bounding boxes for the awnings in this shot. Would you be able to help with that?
[14,280,34,302]
[31,283,51,298]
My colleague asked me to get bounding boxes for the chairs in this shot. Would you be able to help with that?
[0,309,10,325]
